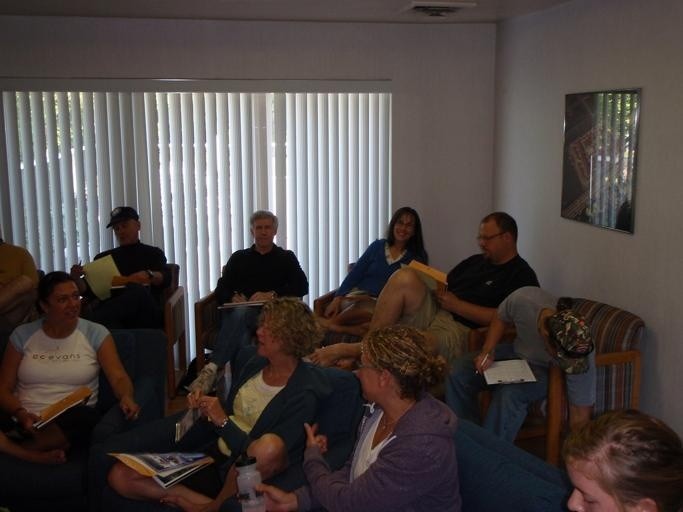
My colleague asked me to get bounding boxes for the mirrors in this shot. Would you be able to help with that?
[561,90,641,234]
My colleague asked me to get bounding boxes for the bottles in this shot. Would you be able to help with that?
[235,454,265,512]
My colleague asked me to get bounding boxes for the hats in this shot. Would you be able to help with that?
[106,207,138,228]
[547,308,594,375]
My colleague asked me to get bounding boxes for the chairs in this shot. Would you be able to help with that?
[163,265,187,397]
[472,295,645,467]
[313,289,367,346]
[192,290,217,372]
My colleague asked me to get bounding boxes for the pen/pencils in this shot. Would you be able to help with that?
[182,385,194,396]
[474,349,492,375]
[78,260,81,266]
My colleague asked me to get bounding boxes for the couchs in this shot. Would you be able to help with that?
[149,408,574,512]
[0,329,163,510]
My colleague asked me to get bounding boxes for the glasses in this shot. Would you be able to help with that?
[478,232,505,241]
[353,359,382,370]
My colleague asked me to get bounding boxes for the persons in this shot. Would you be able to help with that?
[0,208,682,512]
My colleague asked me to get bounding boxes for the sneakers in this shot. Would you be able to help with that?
[188,363,219,396]
